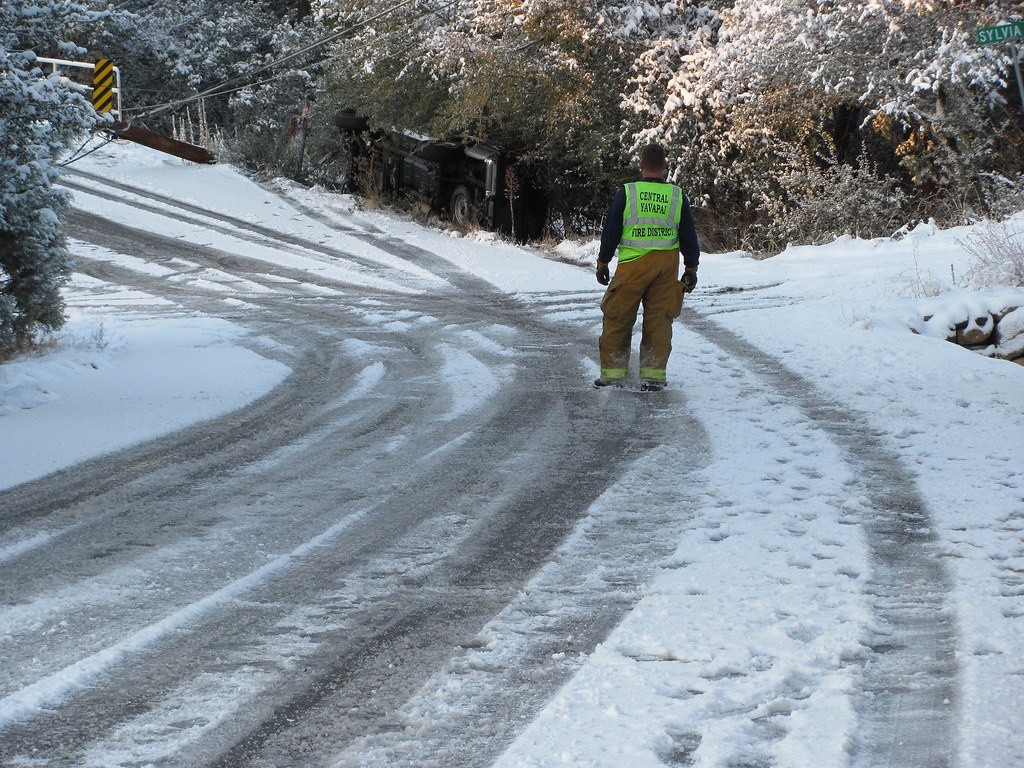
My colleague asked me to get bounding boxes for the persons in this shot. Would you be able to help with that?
[592,144,701,391]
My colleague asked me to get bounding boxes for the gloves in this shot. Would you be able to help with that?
[681,266,698,293]
[596,258,609,285]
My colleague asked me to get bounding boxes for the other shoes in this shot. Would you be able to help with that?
[640,381,667,392]
[594,379,622,389]
